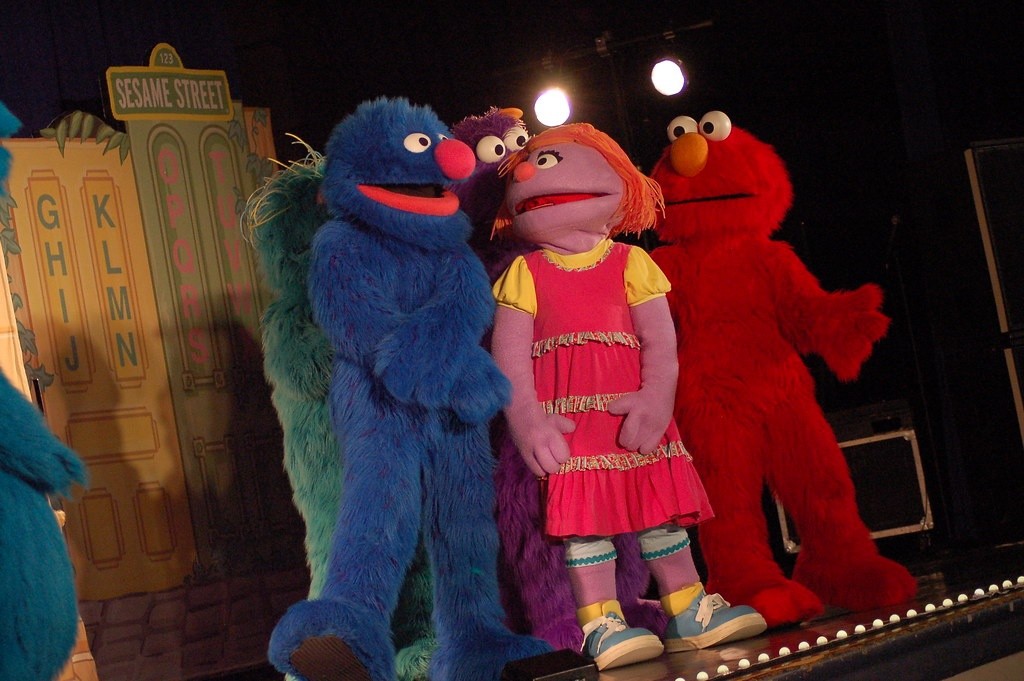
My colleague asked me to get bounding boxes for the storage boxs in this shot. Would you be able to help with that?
[770,399,935,554]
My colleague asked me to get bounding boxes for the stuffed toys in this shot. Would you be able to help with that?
[247,131,439,676]
[623,109,915,630]
[443,111,671,662]
[0,367,97,680]
[266,92,558,680]
[487,121,773,668]
